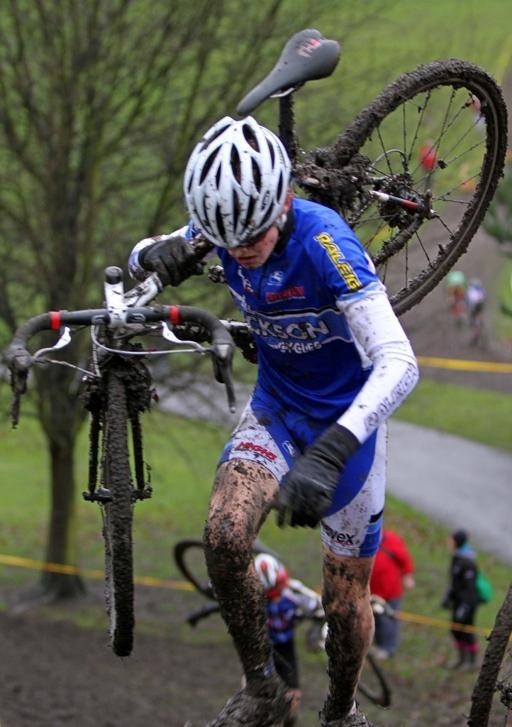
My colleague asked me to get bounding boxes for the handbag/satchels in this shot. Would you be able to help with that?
[474,572,492,605]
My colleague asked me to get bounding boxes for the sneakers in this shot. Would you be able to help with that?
[447,660,482,674]
[318,710,372,726]
[206,670,299,727]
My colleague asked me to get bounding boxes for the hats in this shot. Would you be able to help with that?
[452,528,466,547]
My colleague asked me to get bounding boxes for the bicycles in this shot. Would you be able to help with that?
[462,584,512,727]
[444,300,491,353]
[5,23,510,658]
[173,529,392,709]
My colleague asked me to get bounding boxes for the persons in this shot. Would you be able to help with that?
[446,267,466,333]
[441,532,492,669]
[370,527,417,661]
[254,551,322,711]
[462,277,485,349]
[127,121,421,727]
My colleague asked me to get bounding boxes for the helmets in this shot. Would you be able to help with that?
[179,114,296,252]
[248,548,288,600]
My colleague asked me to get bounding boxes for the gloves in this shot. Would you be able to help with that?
[143,234,206,290]
[269,437,352,531]
